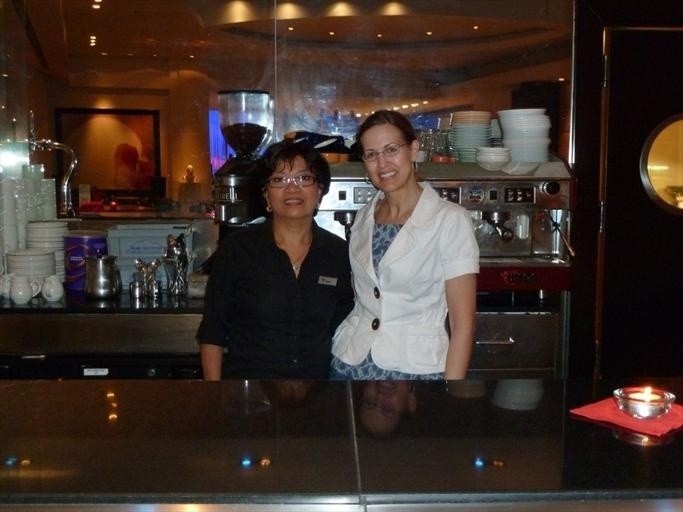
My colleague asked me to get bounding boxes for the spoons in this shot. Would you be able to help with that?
[133,256,158,294]
[162,233,187,293]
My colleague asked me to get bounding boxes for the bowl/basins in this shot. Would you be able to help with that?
[612,387,675,419]
[619,432,671,447]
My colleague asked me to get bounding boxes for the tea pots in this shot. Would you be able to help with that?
[86,252,122,301]
[9,275,40,305]
[43,275,63,303]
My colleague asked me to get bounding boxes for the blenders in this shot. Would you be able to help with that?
[210,89,270,229]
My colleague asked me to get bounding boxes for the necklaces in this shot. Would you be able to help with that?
[273,235,310,272]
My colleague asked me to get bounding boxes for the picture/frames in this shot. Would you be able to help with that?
[54,107,160,195]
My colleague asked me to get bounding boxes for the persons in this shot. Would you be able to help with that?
[329,109,479,379]
[196,142,354,381]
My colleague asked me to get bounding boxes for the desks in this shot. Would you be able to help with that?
[0,287,230,354]
[0,380,683,507]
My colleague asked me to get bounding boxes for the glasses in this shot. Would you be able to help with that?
[361,141,409,162]
[264,176,319,187]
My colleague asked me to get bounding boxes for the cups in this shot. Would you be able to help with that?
[164,263,188,294]
[133,271,157,297]
[1,163,56,252]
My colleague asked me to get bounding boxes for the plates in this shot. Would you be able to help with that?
[492,379,545,412]
[25,221,71,283]
[449,110,491,160]
[5,249,56,279]
[502,162,541,175]
[446,379,485,399]
[475,145,510,161]
[497,108,551,161]
[476,163,506,170]
